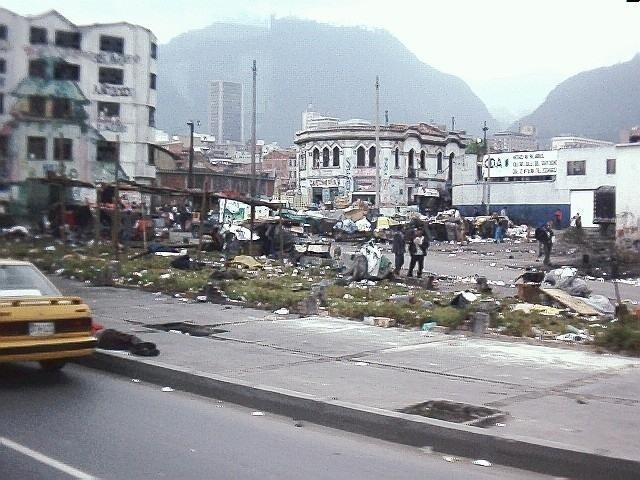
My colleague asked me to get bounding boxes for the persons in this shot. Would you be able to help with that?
[538,222,556,267]
[90,321,161,358]
[393,223,406,275]
[500,207,508,216]
[494,221,503,245]
[554,208,563,230]
[490,212,499,222]
[536,223,545,258]
[405,227,430,278]
[573,212,583,227]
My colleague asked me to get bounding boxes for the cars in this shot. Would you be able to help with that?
[0,259,99,373]
